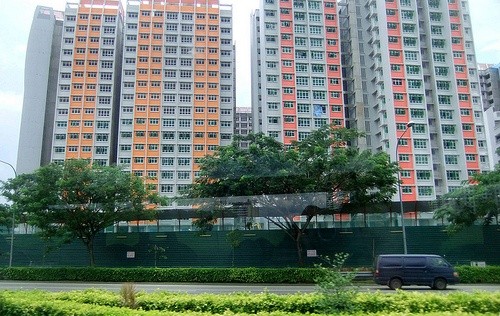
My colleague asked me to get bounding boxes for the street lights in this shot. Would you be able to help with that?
[395,120,417,255]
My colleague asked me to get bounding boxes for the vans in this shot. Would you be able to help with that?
[374,253,455,290]
